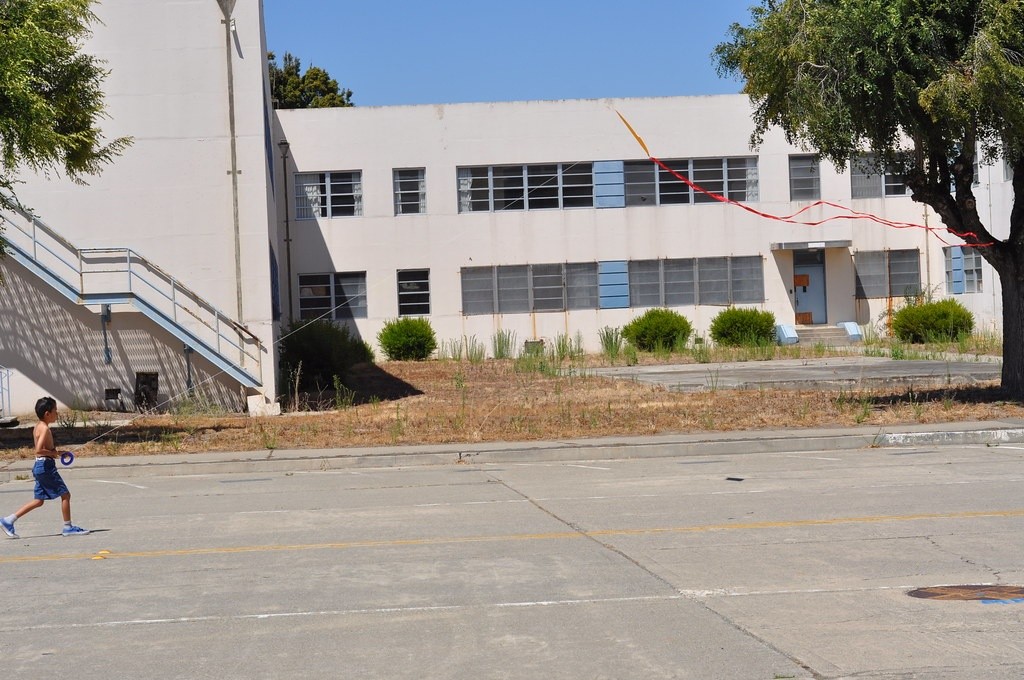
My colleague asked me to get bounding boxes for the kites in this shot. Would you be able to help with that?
[616,111,994,248]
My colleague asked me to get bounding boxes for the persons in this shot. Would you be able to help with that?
[0,396,91,539]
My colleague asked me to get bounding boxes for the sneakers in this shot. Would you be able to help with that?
[0,518,19,538]
[62,526,90,536]
